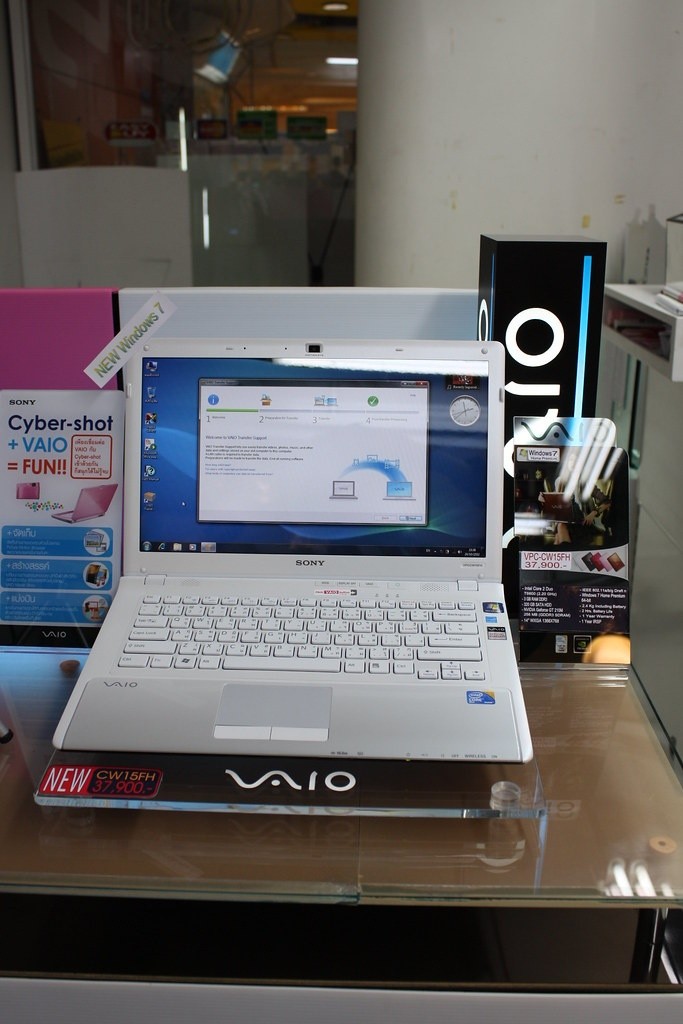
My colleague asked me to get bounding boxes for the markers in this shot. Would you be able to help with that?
[661,286,683,304]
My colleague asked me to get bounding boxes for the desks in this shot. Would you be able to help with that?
[0,647,683,995]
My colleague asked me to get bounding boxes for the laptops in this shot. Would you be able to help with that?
[383,481,416,501]
[51,484,118,523]
[53,338,535,765]
[329,481,358,499]
[541,492,576,523]
